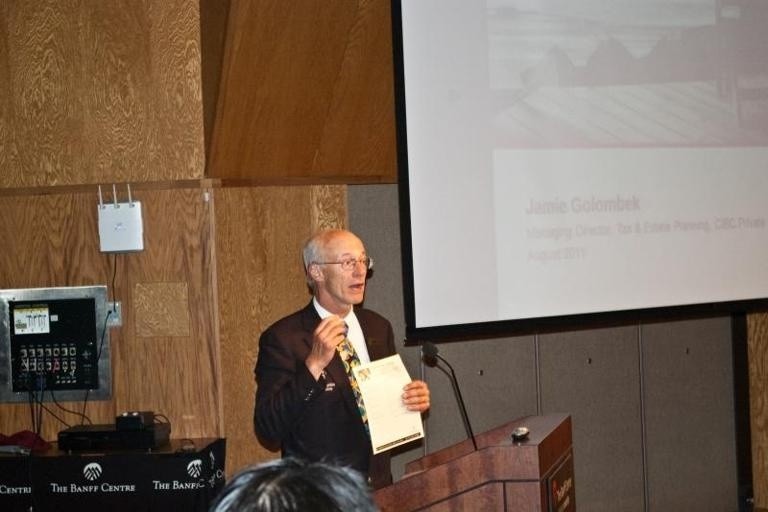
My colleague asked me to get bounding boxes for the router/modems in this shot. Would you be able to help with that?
[95,184,145,254]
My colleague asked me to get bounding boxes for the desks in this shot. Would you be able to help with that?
[0,432,228,512]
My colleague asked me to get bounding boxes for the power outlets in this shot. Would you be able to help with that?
[106,301,123,327]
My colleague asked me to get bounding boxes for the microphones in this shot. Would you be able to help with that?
[422,342,479,454]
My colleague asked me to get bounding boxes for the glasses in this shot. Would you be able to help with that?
[312,256,371,272]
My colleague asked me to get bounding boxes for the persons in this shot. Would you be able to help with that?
[208,453,381,511]
[252,228,431,493]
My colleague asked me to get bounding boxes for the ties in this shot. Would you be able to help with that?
[332,322,371,441]
